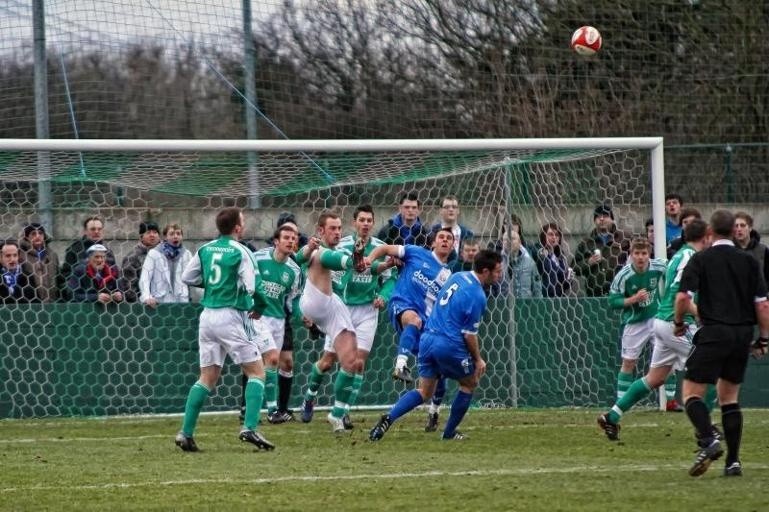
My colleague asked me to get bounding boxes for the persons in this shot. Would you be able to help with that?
[362,227,455,431]
[238,196,768,304]
[175,208,275,450]
[598,220,725,442]
[608,238,686,415]
[241,225,313,426]
[238,219,320,423]
[672,211,769,477]
[0,215,204,309]
[370,250,502,442]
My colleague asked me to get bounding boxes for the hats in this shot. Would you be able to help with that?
[139,220,160,237]
[85,244,108,254]
[25,222,46,239]
[594,205,614,223]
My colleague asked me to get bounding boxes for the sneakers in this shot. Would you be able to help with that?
[238,426,275,451]
[441,431,471,441]
[723,460,743,476]
[342,414,354,430]
[266,409,285,425]
[695,424,726,441]
[300,398,315,423]
[239,414,263,426]
[597,411,621,442]
[277,410,297,422]
[351,236,366,274]
[391,366,415,383]
[175,432,198,452]
[327,411,345,433]
[369,414,393,441]
[425,411,440,432]
[688,439,725,477]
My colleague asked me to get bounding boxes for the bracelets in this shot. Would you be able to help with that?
[673,320,684,326]
[754,336,768,349]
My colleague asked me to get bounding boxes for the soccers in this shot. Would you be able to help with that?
[573,24,605,60]
